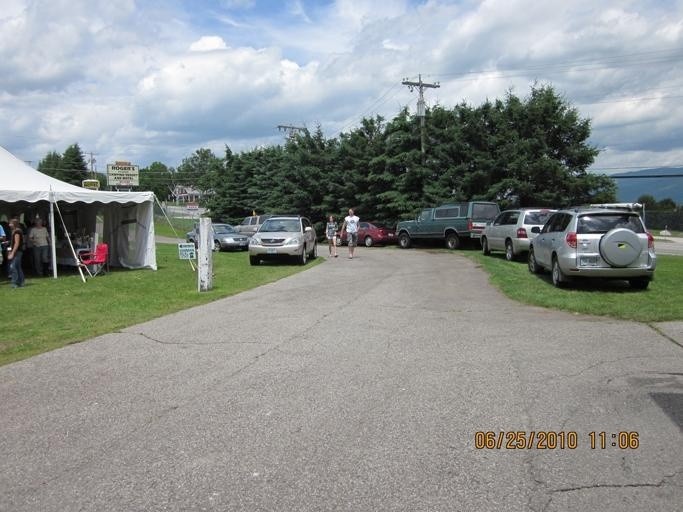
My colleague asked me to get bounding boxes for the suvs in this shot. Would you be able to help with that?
[395,201,500,250]
[527,208,656,291]
[233,215,271,238]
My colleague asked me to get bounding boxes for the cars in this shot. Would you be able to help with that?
[248,217,318,265]
[479,209,559,261]
[324,222,396,247]
[185,224,249,252]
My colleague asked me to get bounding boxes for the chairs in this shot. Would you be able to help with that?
[79,243,109,277]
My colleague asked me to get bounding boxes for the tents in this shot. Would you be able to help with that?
[0,144,158,273]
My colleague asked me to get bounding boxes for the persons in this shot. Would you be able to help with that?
[8,219,25,287]
[326,216,339,257]
[1,224,7,264]
[340,209,361,258]
[30,218,52,275]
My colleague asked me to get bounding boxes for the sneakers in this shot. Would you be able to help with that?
[334,254,339,257]
[349,256,352,259]
[329,254,332,257]
